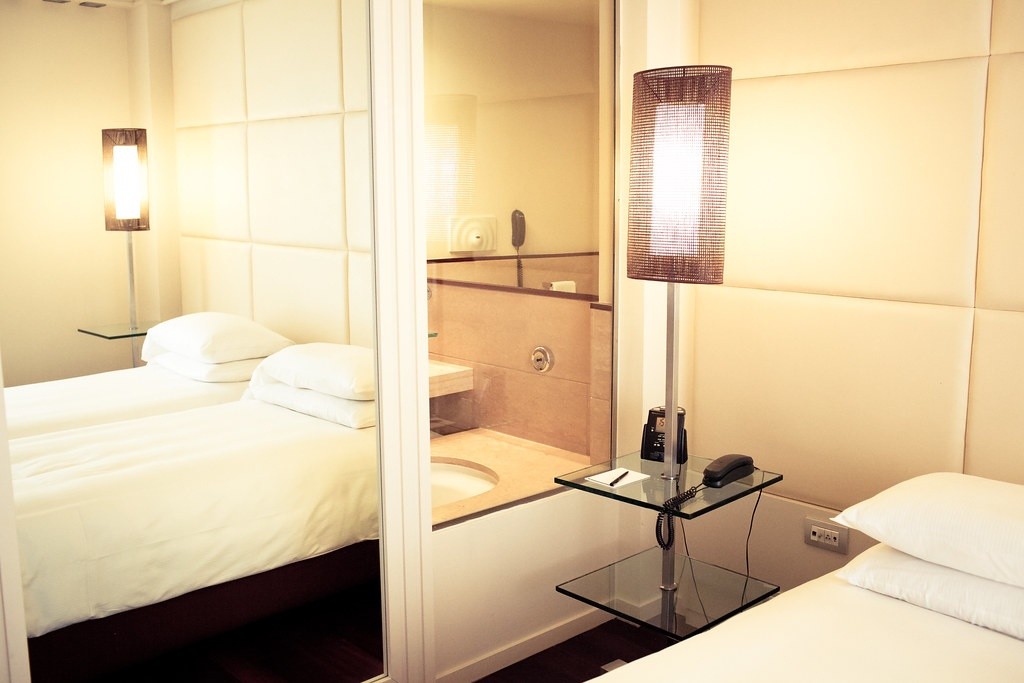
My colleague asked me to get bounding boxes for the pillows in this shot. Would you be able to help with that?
[245,380,375,429]
[828,470,1024,590]
[142,311,296,363]
[147,356,265,381]
[832,541,1024,642]
[251,342,376,400]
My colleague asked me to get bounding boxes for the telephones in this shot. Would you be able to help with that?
[702,453,754,488]
[513,208,525,247]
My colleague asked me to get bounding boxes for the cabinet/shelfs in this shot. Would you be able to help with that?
[554,447,783,640]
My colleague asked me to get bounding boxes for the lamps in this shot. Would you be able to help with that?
[77,126,161,369]
[554,64,779,642]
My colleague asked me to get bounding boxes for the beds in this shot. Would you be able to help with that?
[598,540,1024,683]
[2,310,383,682]
